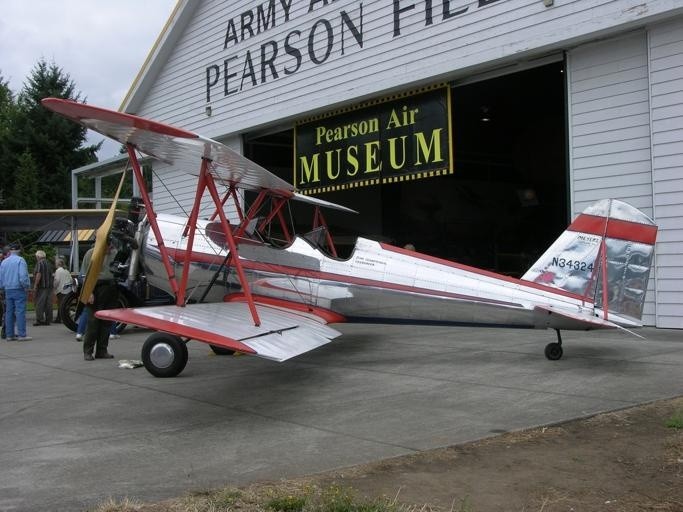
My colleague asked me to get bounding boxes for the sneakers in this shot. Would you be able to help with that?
[1,328,6,339]
[18,336,32,341]
[109,334,121,340]
[76,334,83,342]
[6,335,18,341]
[33,315,63,326]
[94,352,114,359]
[84,353,94,361]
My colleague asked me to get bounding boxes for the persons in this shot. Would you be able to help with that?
[52,259,73,323]
[0,242,31,341]
[32,250,53,325]
[76,236,120,360]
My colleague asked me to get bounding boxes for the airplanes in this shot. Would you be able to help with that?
[41,97,659,378]
